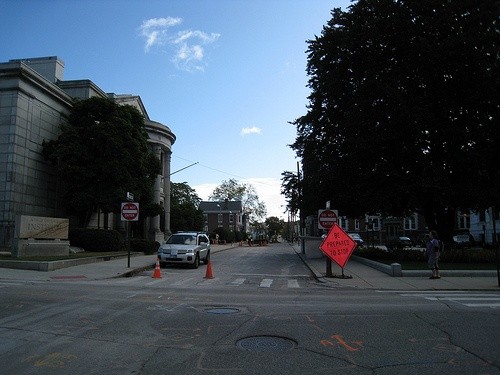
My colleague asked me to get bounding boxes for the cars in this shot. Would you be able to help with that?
[398,237,411,248]
[347,234,364,248]
[421,234,431,247]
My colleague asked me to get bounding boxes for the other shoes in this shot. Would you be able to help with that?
[430,276,436,279]
[437,277,440,278]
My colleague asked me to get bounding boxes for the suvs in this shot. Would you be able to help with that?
[453,235,471,249]
[158,231,211,268]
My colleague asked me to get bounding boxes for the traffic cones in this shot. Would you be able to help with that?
[151,258,162,278]
[203,260,214,278]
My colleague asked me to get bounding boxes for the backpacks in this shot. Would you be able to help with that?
[434,238,444,252]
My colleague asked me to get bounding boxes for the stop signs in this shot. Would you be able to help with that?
[121,202,139,221]
[318,210,338,229]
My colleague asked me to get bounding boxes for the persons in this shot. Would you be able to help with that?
[426,231,441,279]
[247,234,253,247]
[208,232,219,244]
